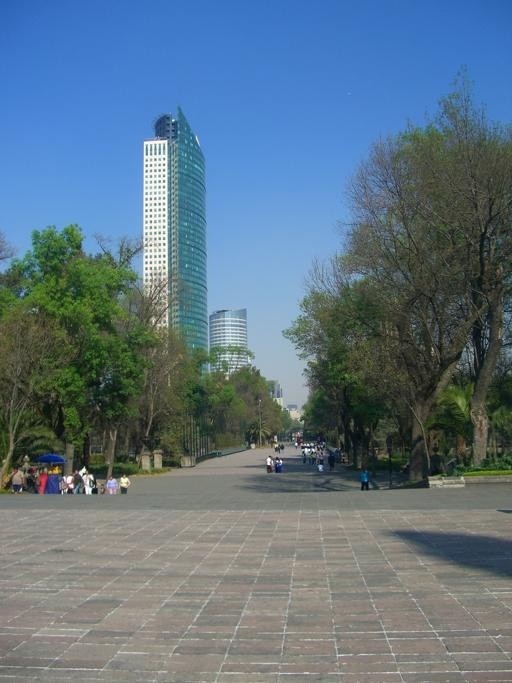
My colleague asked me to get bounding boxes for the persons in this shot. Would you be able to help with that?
[361,470,369,490]
[10,464,131,495]
[266,442,335,473]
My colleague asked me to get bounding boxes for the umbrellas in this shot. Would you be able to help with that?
[33,456,66,466]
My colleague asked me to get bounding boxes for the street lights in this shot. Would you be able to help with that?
[259,400,261,448]
